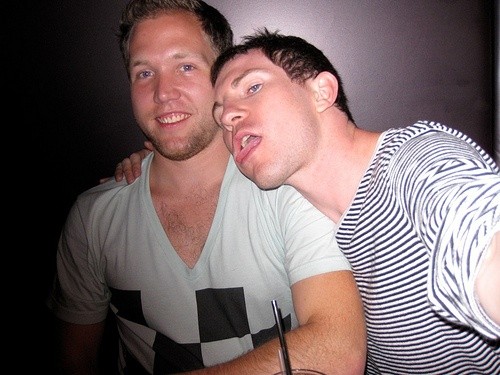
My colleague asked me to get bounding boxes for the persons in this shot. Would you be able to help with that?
[100,26,500,375]
[51,0,368,375]
